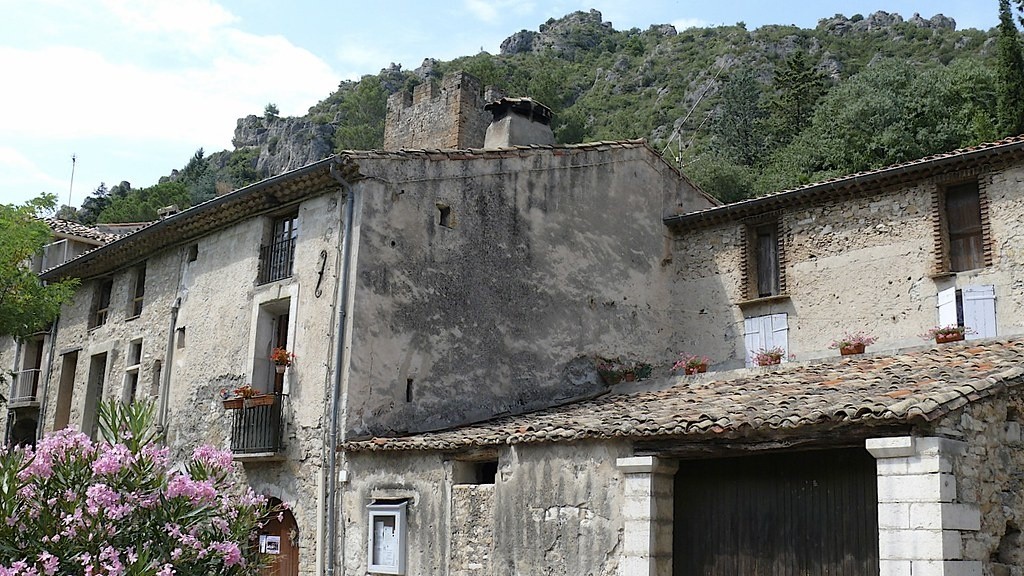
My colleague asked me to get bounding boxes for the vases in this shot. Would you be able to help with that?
[936,333,961,343]
[758,356,781,365]
[275,365,286,373]
[685,364,707,375]
[243,394,274,408]
[602,373,633,383]
[839,344,865,355]
[222,399,243,409]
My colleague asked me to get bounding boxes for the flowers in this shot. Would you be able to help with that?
[827,330,880,351]
[917,324,978,341]
[270,345,295,367]
[597,357,656,383]
[669,350,710,375]
[233,383,260,397]
[751,347,797,366]
